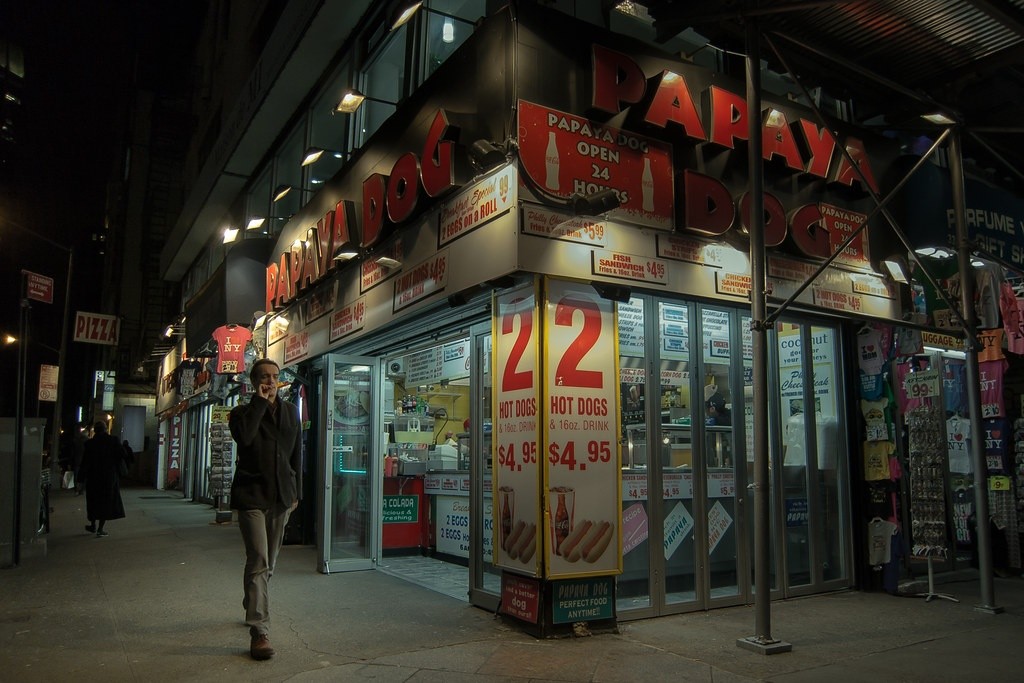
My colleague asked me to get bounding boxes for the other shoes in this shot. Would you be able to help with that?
[98,531,110,537]
[85,525,96,533]
[251,634,275,660]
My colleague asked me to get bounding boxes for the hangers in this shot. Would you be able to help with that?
[186,322,237,362]
[856,247,1023,523]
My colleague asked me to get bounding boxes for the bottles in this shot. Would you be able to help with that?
[501,494,511,548]
[384,453,392,477]
[392,452,398,476]
[397,398,402,415]
[425,400,429,416]
[407,394,412,413]
[416,397,421,413]
[553,495,569,555]
[403,396,407,414]
[421,397,425,415]
[545,132,560,190]
[642,158,654,212]
[412,396,416,413]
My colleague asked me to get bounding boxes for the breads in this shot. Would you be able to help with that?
[504,520,537,563]
[560,520,614,562]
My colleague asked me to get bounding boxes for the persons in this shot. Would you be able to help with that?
[123,440,135,465]
[72,431,90,496]
[705,384,731,448]
[229,359,302,656]
[80,421,129,537]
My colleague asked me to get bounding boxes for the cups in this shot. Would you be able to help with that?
[497,489,514,550]
[549,490,574,557]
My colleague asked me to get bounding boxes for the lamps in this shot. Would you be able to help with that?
[300,146,358,167]
[250,310,266,330]
[222,223,280,246]
[885,254,913,284]
[335,89,397,114]
[245,214,293,231]
[332,241,364,260]
[388,0,487,40]
[468,139,507,173]
[272,184,321,200]
[582,188,618,216]
